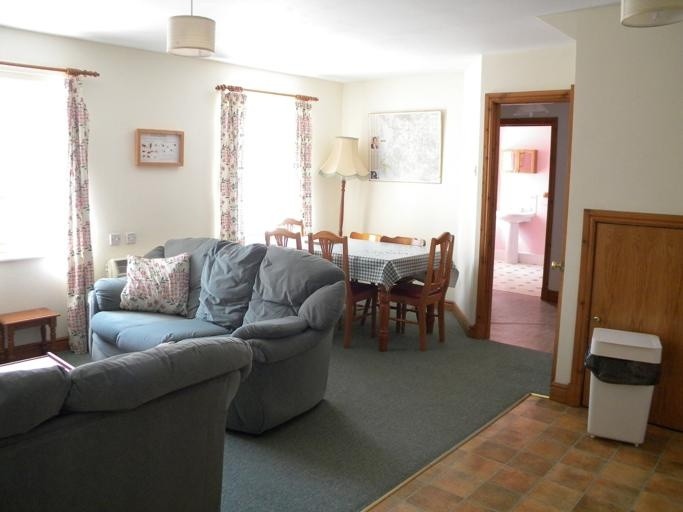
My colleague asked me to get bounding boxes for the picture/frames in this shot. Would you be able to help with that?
[134,128,184,168]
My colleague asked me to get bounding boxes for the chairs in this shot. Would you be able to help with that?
[262,216,459,354]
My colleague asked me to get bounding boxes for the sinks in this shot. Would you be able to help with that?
[500,210,535,223]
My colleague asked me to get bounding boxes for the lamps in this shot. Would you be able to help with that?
[313,134,369,238]
[166,0,216,60]
[619,0,682,29]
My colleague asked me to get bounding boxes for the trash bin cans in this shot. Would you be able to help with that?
[584,327,662,447]
